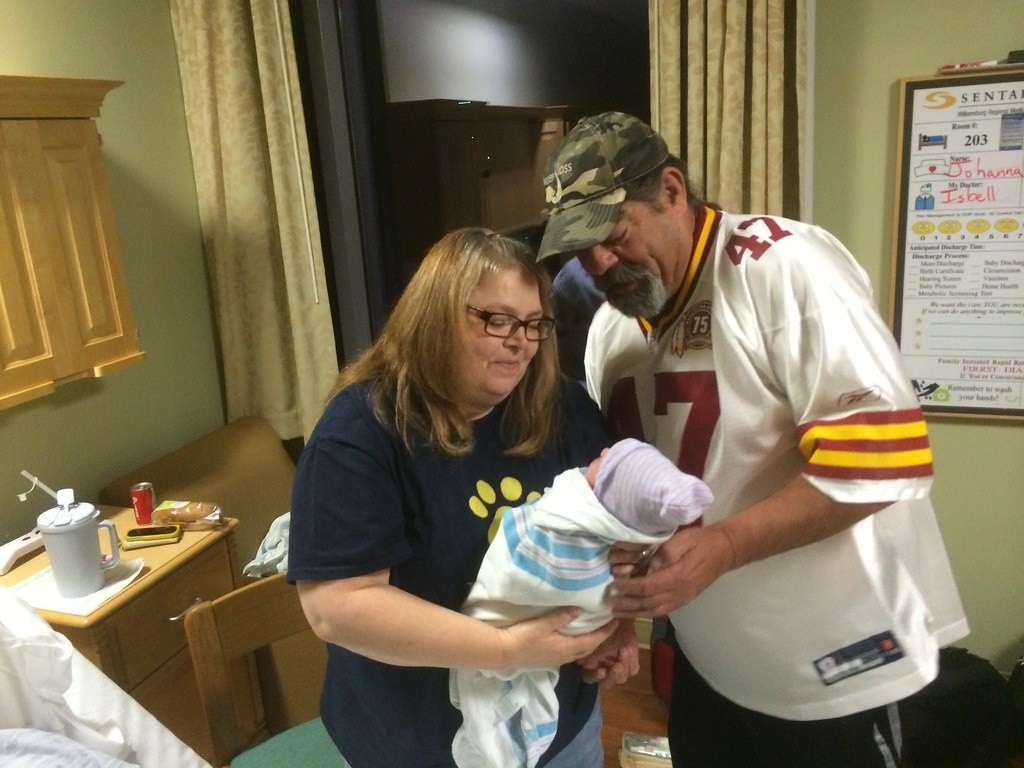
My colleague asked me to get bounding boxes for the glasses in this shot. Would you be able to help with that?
[467,306,555,341]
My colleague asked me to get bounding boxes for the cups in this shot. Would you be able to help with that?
[36,503,119,599]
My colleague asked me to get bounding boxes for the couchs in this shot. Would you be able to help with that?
[99,415,379,739]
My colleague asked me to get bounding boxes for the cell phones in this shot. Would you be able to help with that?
[124,526,182,541]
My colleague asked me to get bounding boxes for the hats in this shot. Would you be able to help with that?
[592,437,715,535]
[534,111,671,264]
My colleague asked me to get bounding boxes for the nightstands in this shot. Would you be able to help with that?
[0,501,273,768]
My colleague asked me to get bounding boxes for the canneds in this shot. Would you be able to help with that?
[130,482,157,524]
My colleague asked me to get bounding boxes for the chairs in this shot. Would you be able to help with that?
[184,570,352,768]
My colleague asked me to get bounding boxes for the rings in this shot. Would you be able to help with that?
[643,551,646,558]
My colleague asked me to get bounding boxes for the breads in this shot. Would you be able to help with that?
[150,500,223,531]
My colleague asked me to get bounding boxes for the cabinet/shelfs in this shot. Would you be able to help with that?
[0,73,148,412]
[388,98,574,300]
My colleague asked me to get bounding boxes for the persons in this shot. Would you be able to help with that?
[285,226,660,768]
[468,436,682,684]
[535,110,972,768]
